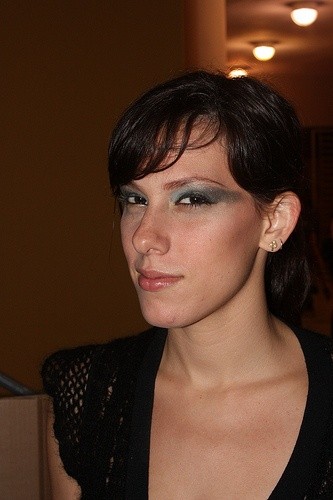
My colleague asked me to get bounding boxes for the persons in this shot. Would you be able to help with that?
[41,69,333,500]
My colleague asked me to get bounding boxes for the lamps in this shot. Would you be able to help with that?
[250,40,279,62]
[287,0,325,27]
